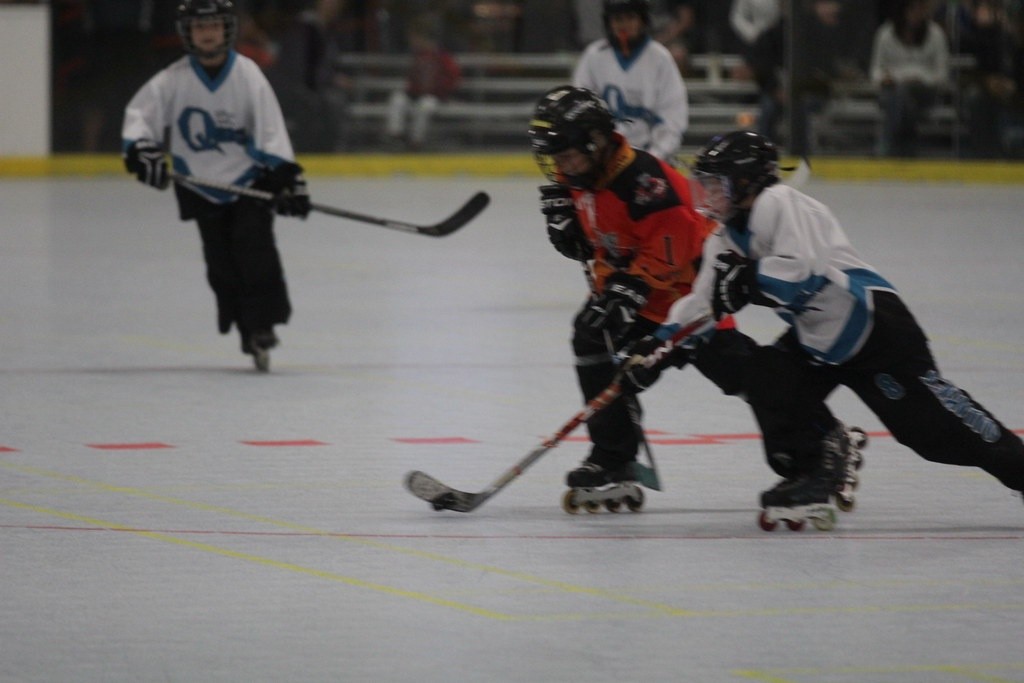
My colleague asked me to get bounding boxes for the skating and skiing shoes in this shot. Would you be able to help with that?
[758,449,840,531]
[813,418,869,511]
[561,461,645,515]
[239,325,278,371]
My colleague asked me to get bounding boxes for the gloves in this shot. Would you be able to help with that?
[571,271,650,357]
[128,139,172,189]
[538,186,595,262]
[613,334,677,400]
[709,251,780,322]
[267,163,309,219]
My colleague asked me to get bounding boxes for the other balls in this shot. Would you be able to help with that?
[431,492,456,512]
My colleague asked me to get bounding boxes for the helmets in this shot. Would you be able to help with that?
[603,0,651,52]
[176,0,239,59]
[527,85,618,194]
[691,130,780,237]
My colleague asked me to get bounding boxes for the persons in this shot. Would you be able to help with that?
[386,14,463,149]
[627,127,1024,530]
[868,1,1024,166]
[120,0,310,371]
[649,0,862,154]
[567,2,688,167]
[527,89,868,512]
[82,0,349,153]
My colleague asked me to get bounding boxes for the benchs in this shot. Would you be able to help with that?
[335,54,967,152]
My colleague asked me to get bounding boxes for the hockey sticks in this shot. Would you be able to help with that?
[167,170,491,239]
[403,310,728,516]
[575,239,663,493]
[648,139,813,190]
